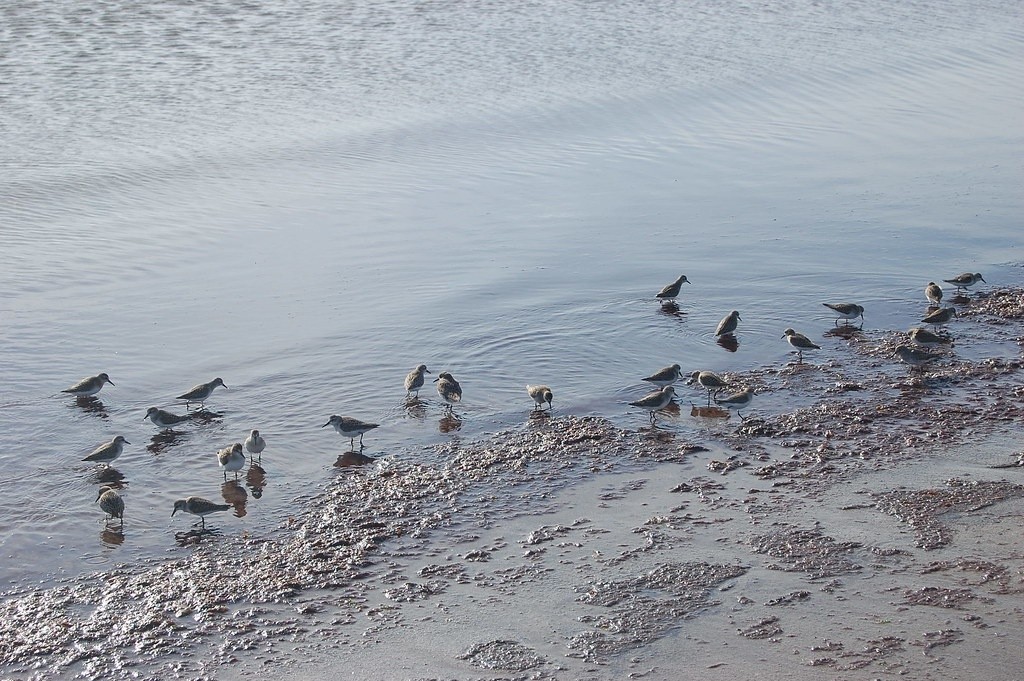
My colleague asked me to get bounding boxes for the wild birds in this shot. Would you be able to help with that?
[714,387,759,420]
[170,497,232,533]
[433,371,462,414]
[687,372,730,409]
[61,372,116,399]
[888,271,991,376]
[781,328,822,365]
[628,386,679,423]
[823,303,866,331]
[639,364,683,391]
[217,429,266,484]
[322,414,380,453]
[712,310,742,339]
[144,377,228,439]
[526,384,554,410]
[404,364,430,400]
[656,275,691,304]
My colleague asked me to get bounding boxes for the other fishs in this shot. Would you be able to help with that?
[81,434,132,526]
